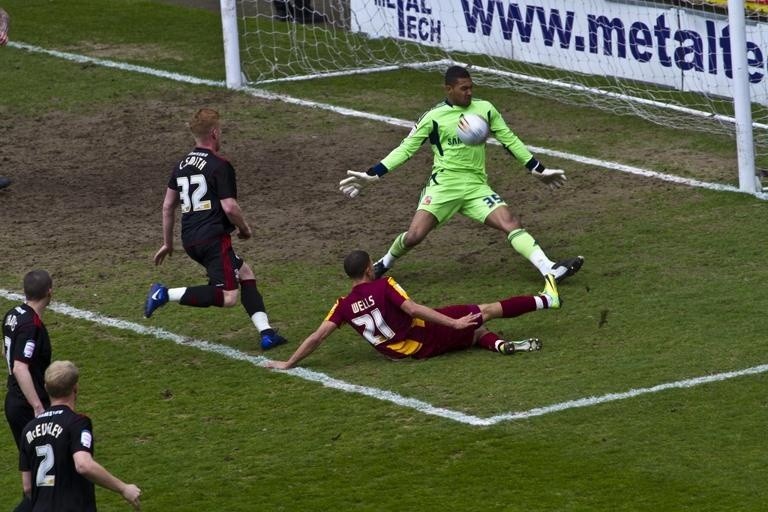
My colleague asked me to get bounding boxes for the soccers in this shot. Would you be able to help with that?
[457,112,490,145]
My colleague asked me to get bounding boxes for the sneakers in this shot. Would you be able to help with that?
[144,281,166,318]
[260,327,288,350]
[373,256,393,280]
[499,337,543,354]
[538,273,561,309]
[544,256,585,285]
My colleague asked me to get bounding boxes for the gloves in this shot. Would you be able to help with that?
[339,166,381,199]
[530,160,568,192]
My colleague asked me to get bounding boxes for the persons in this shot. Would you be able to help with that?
[339,65,584,284]
[12,360,144,512]
[3,269,54,500]
[144,106,291,348]
[263,251,566,369]
